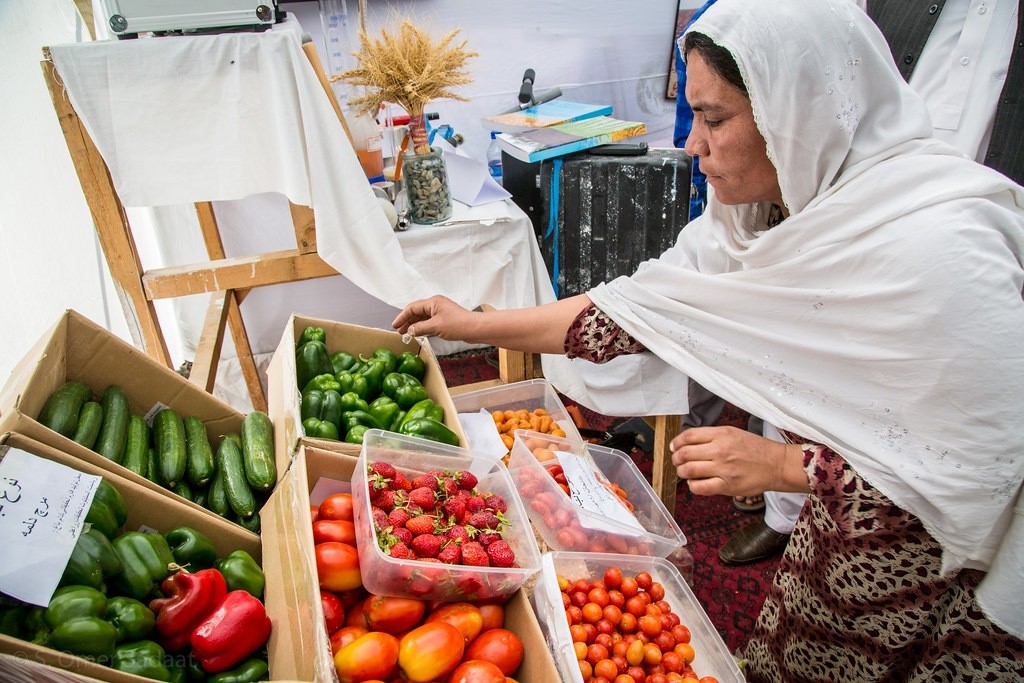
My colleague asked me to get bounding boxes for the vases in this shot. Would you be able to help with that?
[400,147,453,225]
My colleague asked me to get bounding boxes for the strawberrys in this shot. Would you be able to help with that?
[358,461,523,599]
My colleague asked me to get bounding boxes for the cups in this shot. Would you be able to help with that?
[371,182,396,203]
[367,174,385,185]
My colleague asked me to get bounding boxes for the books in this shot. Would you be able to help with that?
[496,116,647,163]
[481,99,613,134]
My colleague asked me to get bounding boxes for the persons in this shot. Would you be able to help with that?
[391,0,1024,683]
[673,0,1024,566]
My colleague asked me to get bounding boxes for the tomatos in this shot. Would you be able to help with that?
[554,566,719,683]
[310,493,525,683]
[519,464,656,557]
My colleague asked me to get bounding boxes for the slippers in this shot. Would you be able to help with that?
[732,487,769,510]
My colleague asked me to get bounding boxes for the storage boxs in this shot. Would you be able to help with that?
[0,310,747,683]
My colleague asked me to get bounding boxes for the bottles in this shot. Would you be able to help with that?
[401,146,453,225]
[345,103,383,178]
[385,113,439,127]
[487,131,503,186]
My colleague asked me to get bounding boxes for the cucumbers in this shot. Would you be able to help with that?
[40,380,278,530]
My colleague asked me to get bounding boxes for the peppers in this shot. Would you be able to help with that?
[0,478,272,683]
[296,325,460,446]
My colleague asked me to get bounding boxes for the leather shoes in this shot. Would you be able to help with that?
[718,518,792,565]
